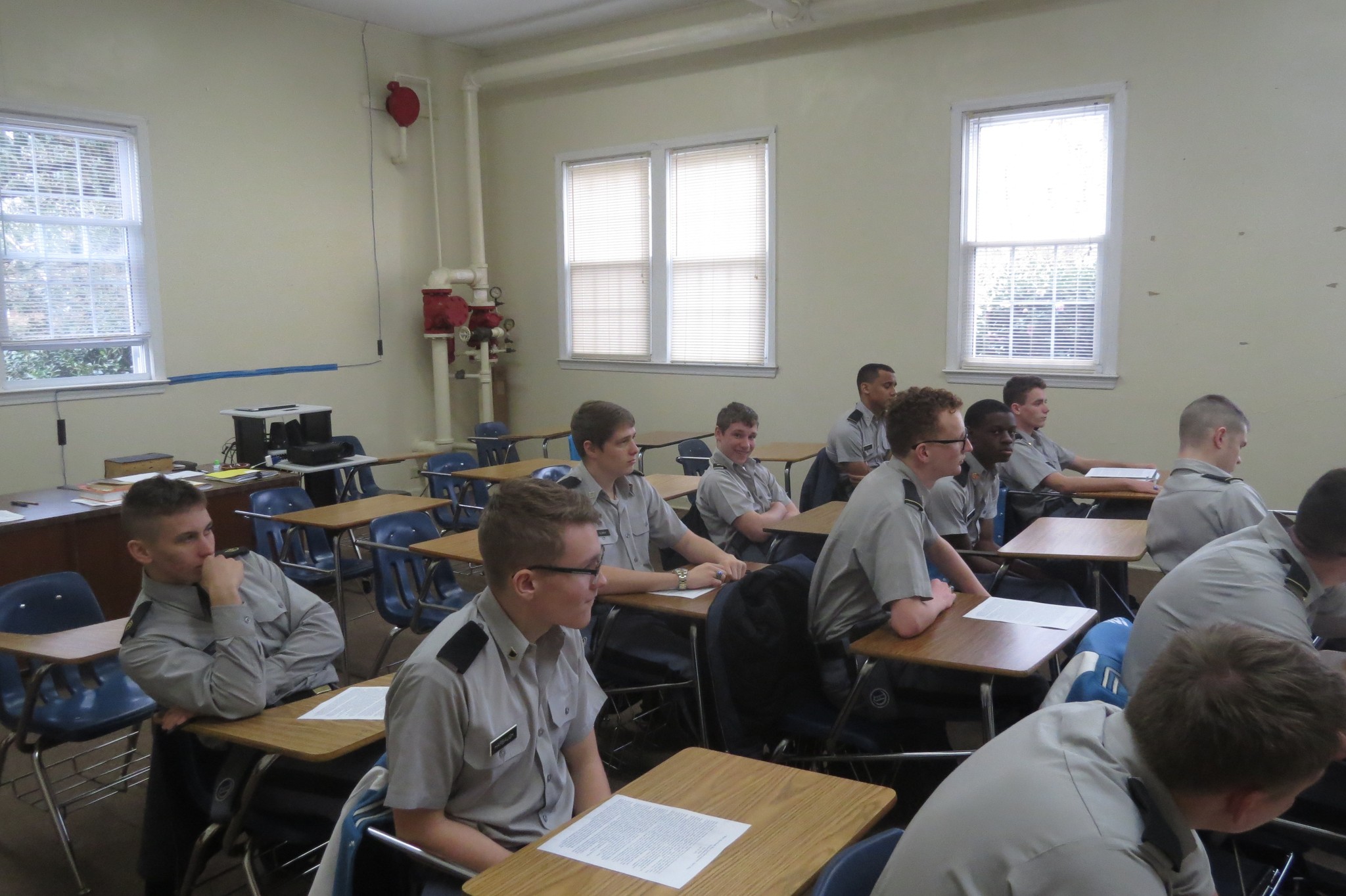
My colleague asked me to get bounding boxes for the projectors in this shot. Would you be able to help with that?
[288,441,355,466]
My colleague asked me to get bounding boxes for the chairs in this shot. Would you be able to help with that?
[0,420,1346,896]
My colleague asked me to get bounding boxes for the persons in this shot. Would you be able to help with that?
[118,476,380,819]
[869,624,1345,896]
[383,478,614,875]
[924,399,1099,659]
[1122,467,1346,843]
[554,400,746,747]
[695,402,819,565]
[996,375,1163,621]
[826,364,898,501]
[1146,393,1269,574]
[808,386,1050,731]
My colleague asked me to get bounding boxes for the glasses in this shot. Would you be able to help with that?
[911,427,968,450]
[511,544,605,585]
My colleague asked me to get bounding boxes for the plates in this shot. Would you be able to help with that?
[221,463,250,471]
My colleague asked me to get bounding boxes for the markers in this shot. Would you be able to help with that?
[10,501,28,507]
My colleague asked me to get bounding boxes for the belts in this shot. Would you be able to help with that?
[264,685,332,709]
[817,637,856,660]
[1041,498,1073,517]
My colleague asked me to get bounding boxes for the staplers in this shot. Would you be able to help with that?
[171,460,197,472]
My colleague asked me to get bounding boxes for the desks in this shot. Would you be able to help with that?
[761,501,853,563]
[366,747,898,896]
[470,425,571,461]
[237,494,452,575]
[957,514,1149,620]
[0,462,300,622]
[263,455,380,591]
[357,530,484,626]
[824,594,1100,762]
[1011,468,1173,519]
[0,615,130,738]
[421,458,580,508]
[595,559,771,750]
[186,671,394,850]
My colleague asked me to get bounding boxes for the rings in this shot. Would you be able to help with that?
[1153,485,1158,490]
[717,571,722,580]
[948,585,954,592]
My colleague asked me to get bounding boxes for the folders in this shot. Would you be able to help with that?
[204,469,280,484]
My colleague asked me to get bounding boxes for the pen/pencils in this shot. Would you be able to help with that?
[16,501,39,505]
[746,569,751,574]
[197,469,209,473]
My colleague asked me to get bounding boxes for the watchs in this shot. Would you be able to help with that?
[672,568,688,590]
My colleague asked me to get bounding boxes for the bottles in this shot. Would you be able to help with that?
[212,460,221,473]
